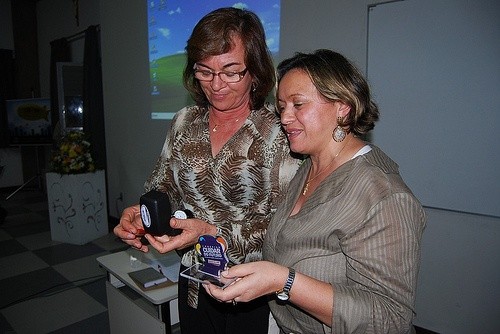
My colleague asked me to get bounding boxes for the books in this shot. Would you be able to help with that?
[129,267,167,288]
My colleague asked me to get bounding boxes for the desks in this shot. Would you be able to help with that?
[46,169,110,245]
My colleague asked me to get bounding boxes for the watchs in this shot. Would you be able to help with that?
[275,267,295,301]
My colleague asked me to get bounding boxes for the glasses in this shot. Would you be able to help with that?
[192,61,248,83]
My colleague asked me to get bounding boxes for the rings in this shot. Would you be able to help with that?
[231,297,237,307]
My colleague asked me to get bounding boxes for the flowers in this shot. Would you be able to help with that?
[52,127,94,174]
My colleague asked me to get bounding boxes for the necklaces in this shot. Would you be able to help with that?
[212,109,249,133]
[302,134,353,195]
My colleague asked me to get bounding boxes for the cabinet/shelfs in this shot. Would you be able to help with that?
[96,251,181,334]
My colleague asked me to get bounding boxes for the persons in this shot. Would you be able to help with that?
[202,49,427,334]
[113,8,309,334]
